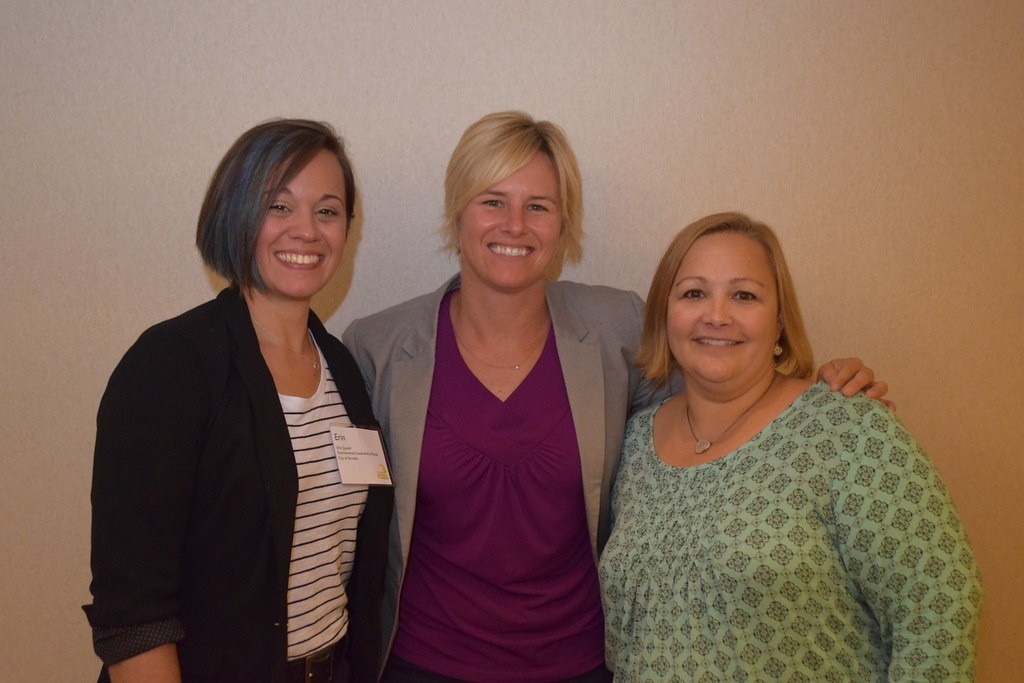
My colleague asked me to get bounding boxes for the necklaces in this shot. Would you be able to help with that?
[686,369,778,455]
[456,307,548,371]
[252,317,320,372]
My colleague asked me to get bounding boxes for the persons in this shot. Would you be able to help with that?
[83,119,399,683]
[341,111,896,683]
[597,211,984,683]
